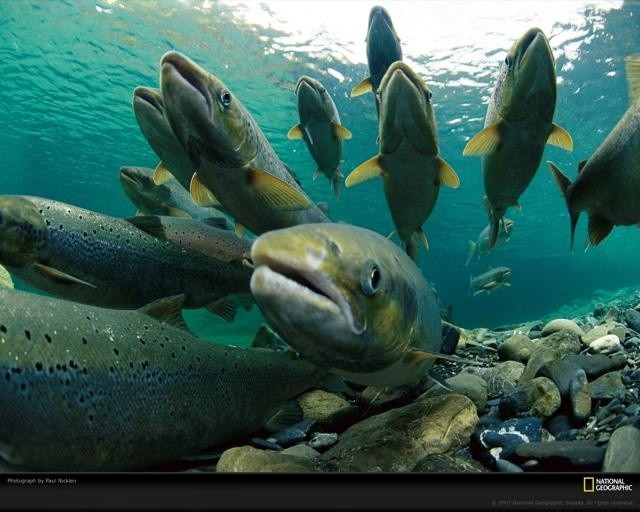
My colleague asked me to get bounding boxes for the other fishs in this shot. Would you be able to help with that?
[250,222,484,391]
